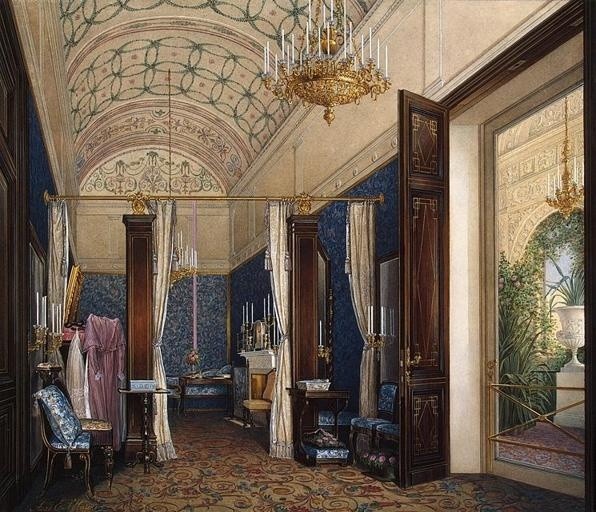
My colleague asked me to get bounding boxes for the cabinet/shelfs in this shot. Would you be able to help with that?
[182,377,232,415]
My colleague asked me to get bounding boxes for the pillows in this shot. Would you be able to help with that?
[33,385,83,469]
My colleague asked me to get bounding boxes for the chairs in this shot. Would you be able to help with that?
[242,368,276,434]
[33,394,94,497]
[55,378,114,487]
[167,376,181,416]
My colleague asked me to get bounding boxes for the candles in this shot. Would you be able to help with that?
[36,292,62,334]
[320,320,322,345]
[371,305,373,334]
[264,294,275,317]
[368,305,371,334]
[243,302,254,324]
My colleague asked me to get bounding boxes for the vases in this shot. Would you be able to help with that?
[190,364,196,375]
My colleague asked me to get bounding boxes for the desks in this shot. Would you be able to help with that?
[286,388,353,467]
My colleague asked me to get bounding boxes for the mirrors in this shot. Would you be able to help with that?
[374,248,400,384]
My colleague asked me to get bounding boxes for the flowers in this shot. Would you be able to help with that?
[185,350,200,366]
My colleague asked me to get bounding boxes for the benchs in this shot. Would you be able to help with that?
[350,381,398,486]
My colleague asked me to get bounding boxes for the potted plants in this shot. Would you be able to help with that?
[545,253,584,372]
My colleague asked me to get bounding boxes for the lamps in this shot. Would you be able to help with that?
[168,68,198,291]
[546,96,584,219]
[261,0,392,127]
